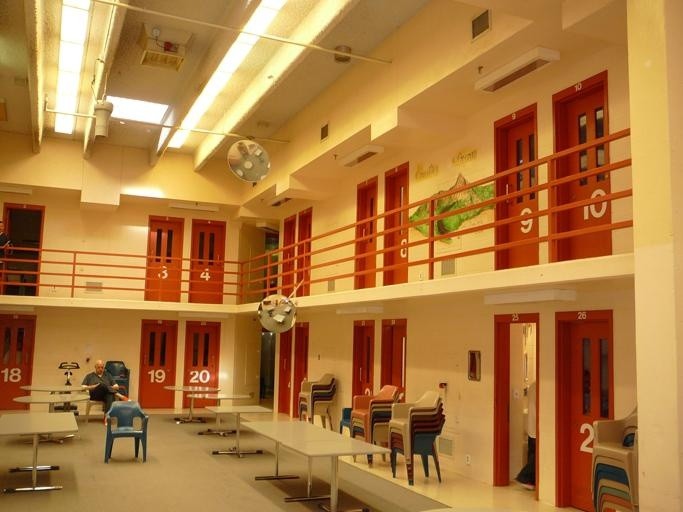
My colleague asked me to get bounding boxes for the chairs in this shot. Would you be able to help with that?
[81,360,149,464]
[592,406,639,512]
[298,373,445,486]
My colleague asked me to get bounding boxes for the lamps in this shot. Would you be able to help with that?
[168,203,219,213]
[341,146,386,168]
[0,187,33,195]
[267,196,292,207]
[474,46,561,95]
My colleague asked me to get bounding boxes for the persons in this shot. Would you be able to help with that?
[515,380,536,490]
[80,360,119,425]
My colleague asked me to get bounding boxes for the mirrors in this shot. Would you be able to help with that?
[226,140,271,183]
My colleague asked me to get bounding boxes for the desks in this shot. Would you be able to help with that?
[12,394,91,444]
[254,148,263,156]
[0,412,78,493]
[164,385,391,512]
[20,385,86,394]
[233,168,244,177]
[243,159,253,169]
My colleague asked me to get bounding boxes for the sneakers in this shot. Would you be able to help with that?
[122,395,133,402]
[104,416,108,427]
